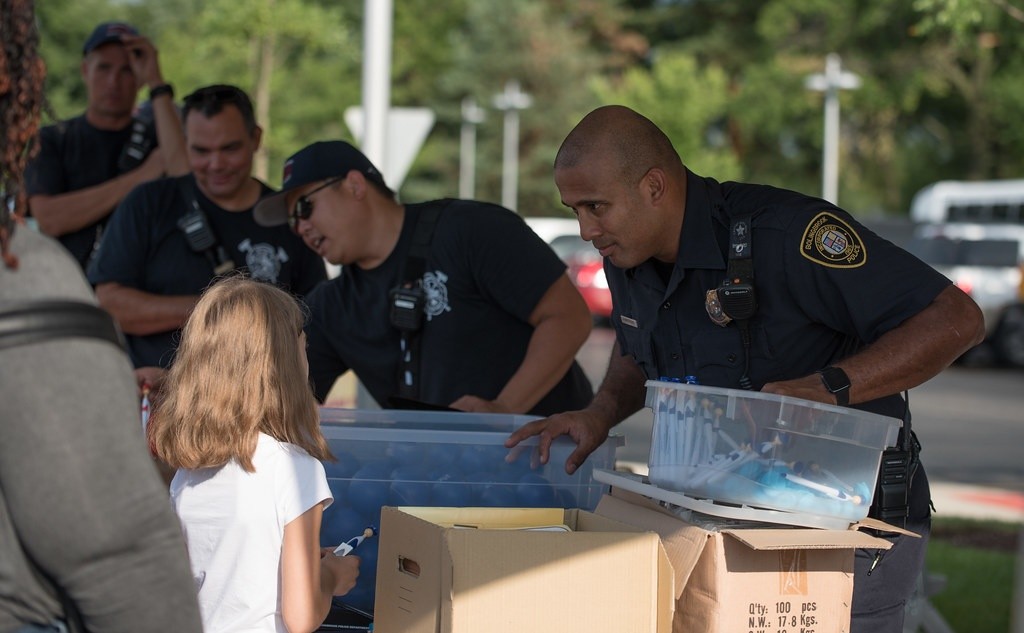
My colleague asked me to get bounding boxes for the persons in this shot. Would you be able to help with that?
[0,0,202,633]
[91,82,329,370]
[504,105,986,633]
[145,271,359,633]
[17,23,192,275]
[133,140,596,419]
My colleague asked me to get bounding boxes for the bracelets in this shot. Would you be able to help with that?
[149,84,174,101]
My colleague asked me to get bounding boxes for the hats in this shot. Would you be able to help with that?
[253,140,368,226]
[84,22,144,58]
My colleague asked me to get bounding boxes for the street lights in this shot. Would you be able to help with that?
[803,71,861,206]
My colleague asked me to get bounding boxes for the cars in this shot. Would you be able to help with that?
[881,220,1024,374]
[548,233,614,325]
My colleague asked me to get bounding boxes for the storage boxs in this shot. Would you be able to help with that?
[374,506,676,633]
[594,485,921,633]
[645,380,904,520]
[312,408,619,616]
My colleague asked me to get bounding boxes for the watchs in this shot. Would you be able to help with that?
[816,365,853,410]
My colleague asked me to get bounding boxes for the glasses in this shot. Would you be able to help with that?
[185,89,243,113]
[290,174,350,231]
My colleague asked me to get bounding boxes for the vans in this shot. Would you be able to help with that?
[915,181,1024,223]
